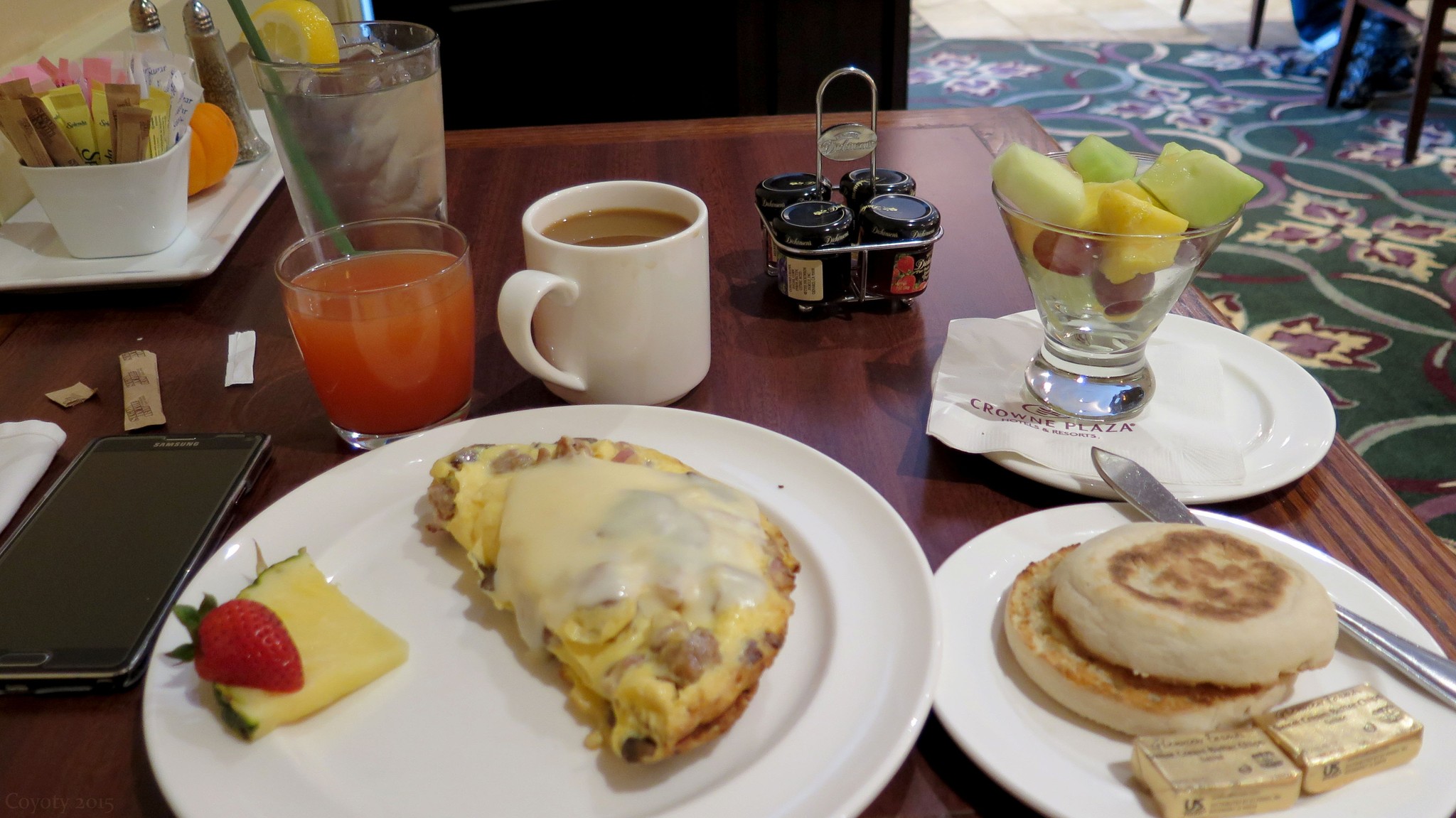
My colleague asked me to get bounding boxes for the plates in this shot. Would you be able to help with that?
[931,308,1335,503]
[0,109,284,289]
[141,401,942,818]
[932,503,1456,818]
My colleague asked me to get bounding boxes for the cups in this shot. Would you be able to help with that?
[990,150,1242,421]
[495,178,710,408]
[276,215,478,450]
[247,20,447,262]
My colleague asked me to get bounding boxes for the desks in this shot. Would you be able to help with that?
[1,103,1456,817]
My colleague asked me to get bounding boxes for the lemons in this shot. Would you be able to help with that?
[240,0,339,76]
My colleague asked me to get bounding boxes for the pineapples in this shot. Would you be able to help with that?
[202,548,409,740]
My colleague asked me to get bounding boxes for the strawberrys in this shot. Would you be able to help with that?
[890,255,926,294]
[165,594,303,693]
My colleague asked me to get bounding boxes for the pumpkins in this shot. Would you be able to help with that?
[186,101,237,195]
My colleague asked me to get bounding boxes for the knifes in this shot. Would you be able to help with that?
[1091,445,1456,710]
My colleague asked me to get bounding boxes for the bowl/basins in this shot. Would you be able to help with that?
[16,127,191,259]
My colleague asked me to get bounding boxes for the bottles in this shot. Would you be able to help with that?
[129,0,173,66]
[754,167,941,302]
[183,0,269,163]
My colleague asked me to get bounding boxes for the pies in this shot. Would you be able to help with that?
[1003,520,1340,736]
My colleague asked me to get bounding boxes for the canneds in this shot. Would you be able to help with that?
[755,167,941,305]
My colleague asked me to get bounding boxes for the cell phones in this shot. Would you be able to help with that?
[0,431,274,698]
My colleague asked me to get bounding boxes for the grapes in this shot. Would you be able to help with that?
[1032,228,1208,315]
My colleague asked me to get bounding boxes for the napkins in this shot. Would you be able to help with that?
[929,311,1174,478]
